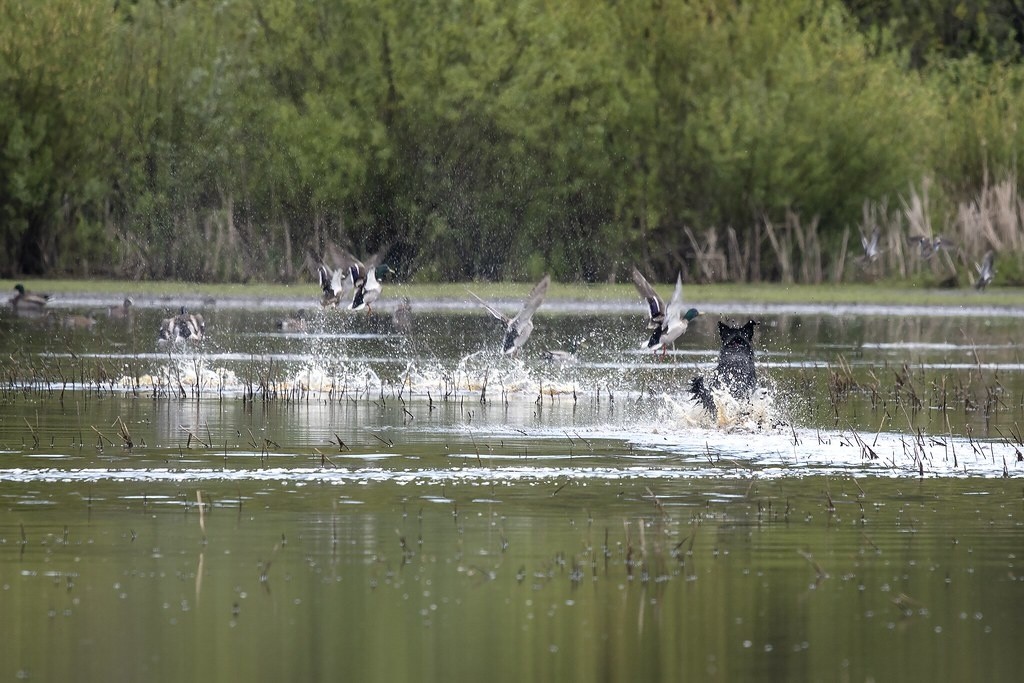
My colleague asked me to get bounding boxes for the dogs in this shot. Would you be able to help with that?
[685,320,757,422]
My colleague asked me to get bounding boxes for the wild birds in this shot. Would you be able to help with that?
[273,234,412,335]
[631,264,706,363]
[467,273,551,358]
[10,283,206,342]
[854,219,995,294]
